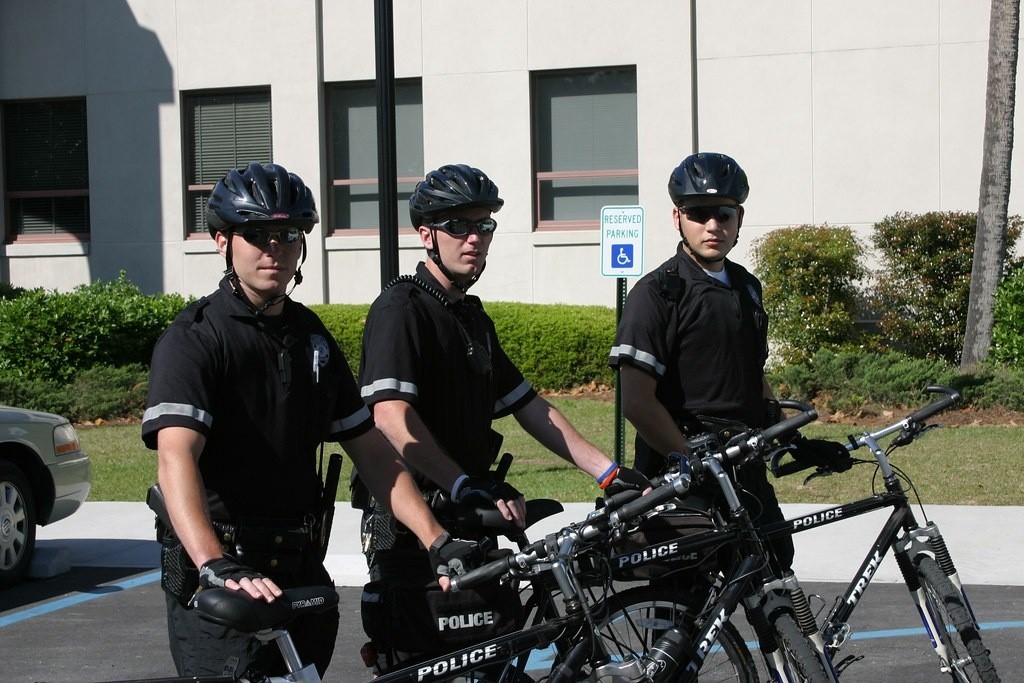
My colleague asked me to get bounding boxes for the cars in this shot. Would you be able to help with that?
[0,405,91,588]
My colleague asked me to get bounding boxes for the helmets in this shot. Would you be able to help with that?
[409,164,504,232]
[668,152,750,208]
[205,162,319,244]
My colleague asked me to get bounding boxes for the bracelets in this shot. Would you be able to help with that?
[597,462,618,483]
[451,475,469,504]
[599,467,621,489]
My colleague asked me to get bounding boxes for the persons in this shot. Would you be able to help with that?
[141,165,478,683]
[350,163,652,583]
[608,154,853,683]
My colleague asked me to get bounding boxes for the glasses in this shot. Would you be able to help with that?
[678,204,741,225]
[424,216,497,237]
[222,226,303,247]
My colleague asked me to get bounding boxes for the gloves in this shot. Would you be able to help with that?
[788,434,851,475]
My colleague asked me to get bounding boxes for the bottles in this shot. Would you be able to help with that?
[640,628,692,681]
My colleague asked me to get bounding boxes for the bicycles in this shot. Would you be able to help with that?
[97,386,1001,683]
